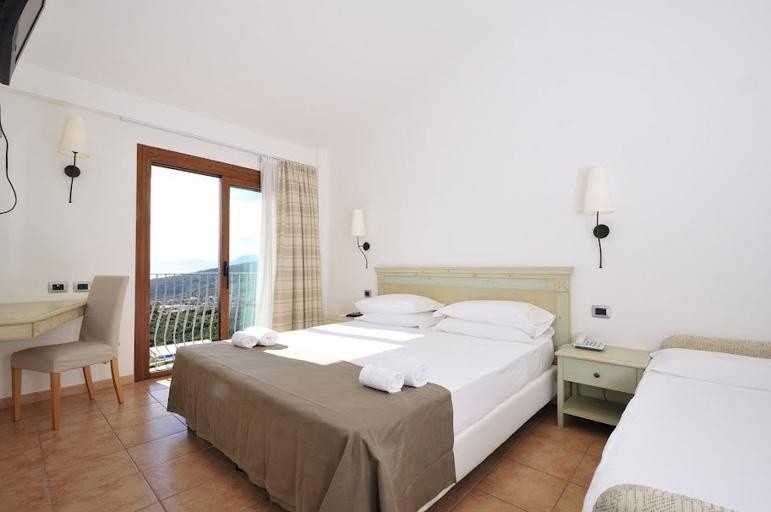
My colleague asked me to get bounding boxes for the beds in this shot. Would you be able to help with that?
[579,334,771,512]
[164,266,571,512]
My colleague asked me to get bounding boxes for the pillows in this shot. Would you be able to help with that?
[647,349,771,390]
[354,293,445,313]
[434,317,555,343]
[354,308,446,328]
[432,299,555,337]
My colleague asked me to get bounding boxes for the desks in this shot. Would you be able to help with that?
[0,299,88,342]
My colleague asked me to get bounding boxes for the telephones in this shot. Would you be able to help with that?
[574,335,606,351]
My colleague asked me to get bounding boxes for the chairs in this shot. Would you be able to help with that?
[10,275,129,430]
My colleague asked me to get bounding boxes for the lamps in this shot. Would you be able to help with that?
[583,166,616,269]
[351,209,370,268]
[58,114,92,202]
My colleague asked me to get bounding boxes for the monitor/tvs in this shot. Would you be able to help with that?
[1,1,45,86]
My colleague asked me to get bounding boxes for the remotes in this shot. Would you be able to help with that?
[346,312,362,317]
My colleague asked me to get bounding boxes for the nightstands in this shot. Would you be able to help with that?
[554,343,653,428]
[326,312,355,323]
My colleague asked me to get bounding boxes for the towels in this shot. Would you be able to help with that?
[386,359,429,387]
[245,325,279,346]
[232,331,258,348]
[358,363,404,393]
